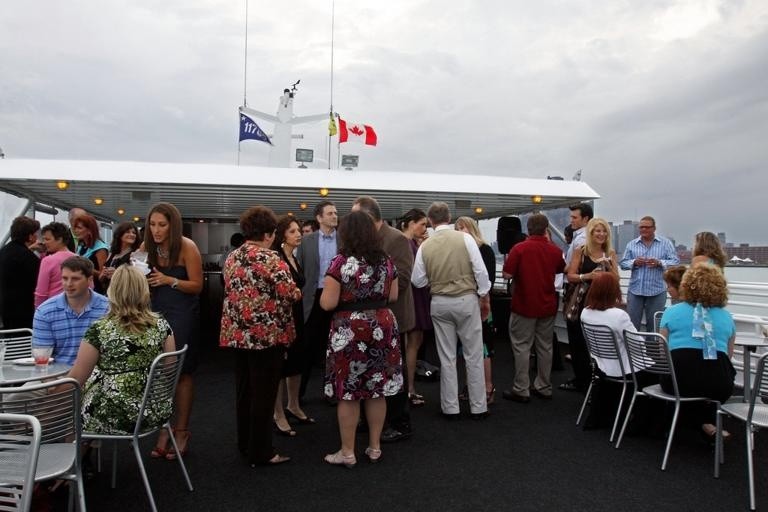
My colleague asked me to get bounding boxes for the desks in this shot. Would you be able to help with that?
[0,356,72,386]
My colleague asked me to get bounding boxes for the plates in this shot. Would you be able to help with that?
[12,357,56,366]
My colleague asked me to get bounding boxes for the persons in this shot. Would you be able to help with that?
[503,214,568,398]
[560,203,737,446]
[2,204,204,471]
[217,197,415,467]
[393,200,499,417]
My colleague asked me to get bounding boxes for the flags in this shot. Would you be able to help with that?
[240,114,271,144]
[338,117,377,146]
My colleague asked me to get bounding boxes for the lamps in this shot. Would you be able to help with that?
[340,154,360,171]
[294,147,314,169]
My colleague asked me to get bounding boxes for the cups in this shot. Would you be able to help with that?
[639,256,645,264]
[0,343,7,369]
[107,266,115,279]
[598,260,607,272]
[756,323,768,338]
[647,257,655,268]
[31,348,53,367]
[131,250,148,269]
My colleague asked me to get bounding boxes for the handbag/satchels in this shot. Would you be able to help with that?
[563,281,590,321]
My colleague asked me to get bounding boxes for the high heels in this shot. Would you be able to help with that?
[284,408,315,424]
[272,415,296,436]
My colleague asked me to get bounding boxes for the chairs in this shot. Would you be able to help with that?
[0,413,44,512]
[0,327,33,361]
[81,344,193,512]
[2,377,86,511]
[575,308,767,511]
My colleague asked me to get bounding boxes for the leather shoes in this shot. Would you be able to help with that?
[531,386,552,400]
[503,390,529,403]
[381,428,416,442]
[560,383,578,390]
[270,454,290,463]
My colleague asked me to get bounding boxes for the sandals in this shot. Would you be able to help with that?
[151,427,191,460]
[365,448,383,463]
[324,450,357,468]
